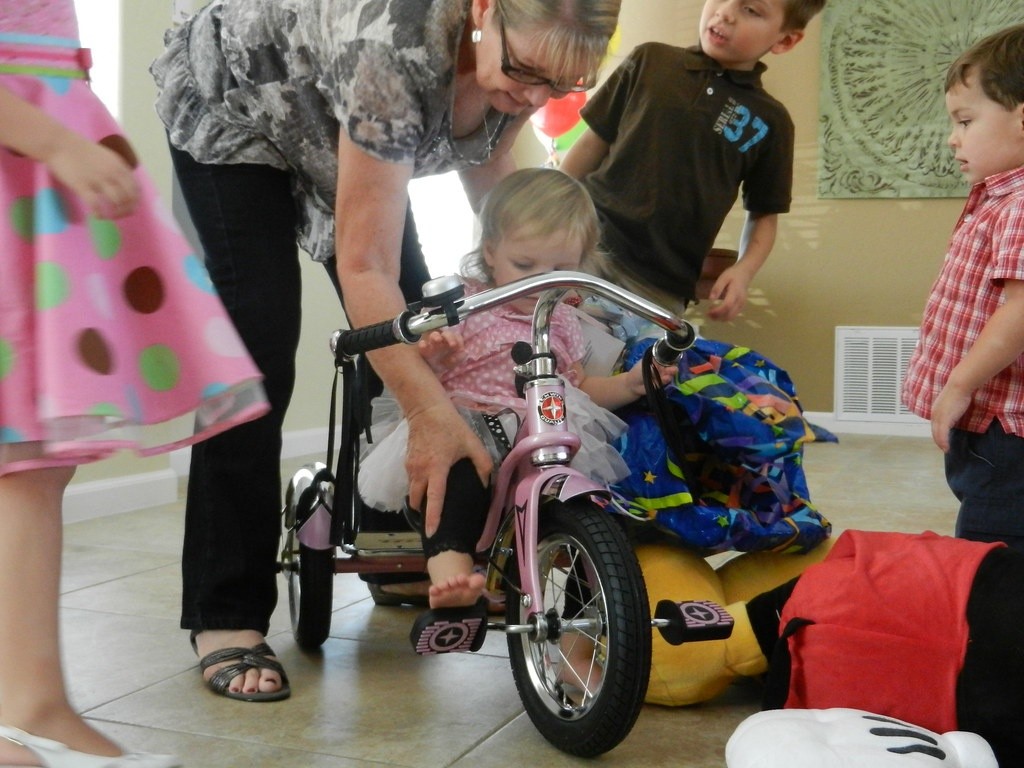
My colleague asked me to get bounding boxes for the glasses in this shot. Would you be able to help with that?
[497,14,598,94]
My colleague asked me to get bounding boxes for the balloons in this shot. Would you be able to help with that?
[527,10,623,151]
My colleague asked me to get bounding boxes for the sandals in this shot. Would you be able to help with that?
[189,629,291,702]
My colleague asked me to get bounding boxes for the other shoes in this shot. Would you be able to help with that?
[0,718,181,768]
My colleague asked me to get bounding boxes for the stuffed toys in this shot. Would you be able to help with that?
[613,528,1024,768]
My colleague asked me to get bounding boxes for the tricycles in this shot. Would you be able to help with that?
[274,272,736,760]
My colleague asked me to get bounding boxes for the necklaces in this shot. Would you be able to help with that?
[470,101,514,163]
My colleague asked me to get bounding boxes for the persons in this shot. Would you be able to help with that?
[897,25,1024,537]
[147,0,622,704]
[354,162,681,701]
[0,1,271,768]
[550,0,826,326]
